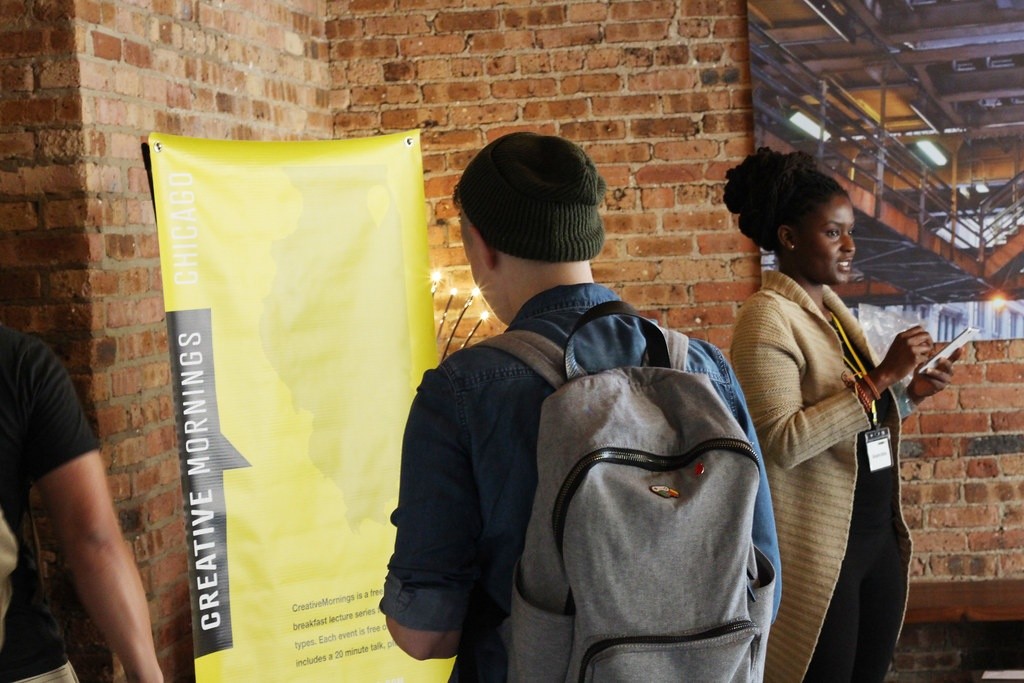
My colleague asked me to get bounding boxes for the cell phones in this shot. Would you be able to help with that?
[919,327,981,375]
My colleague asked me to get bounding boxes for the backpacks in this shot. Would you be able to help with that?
[474,301,774,683]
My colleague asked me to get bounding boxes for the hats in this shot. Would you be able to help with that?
[458,133,606,262]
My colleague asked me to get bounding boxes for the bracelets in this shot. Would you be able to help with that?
[838,369,880,415]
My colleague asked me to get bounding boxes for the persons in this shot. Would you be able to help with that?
[722,146,965,683]
[378,132,782,683]
[0,325,164,683]
